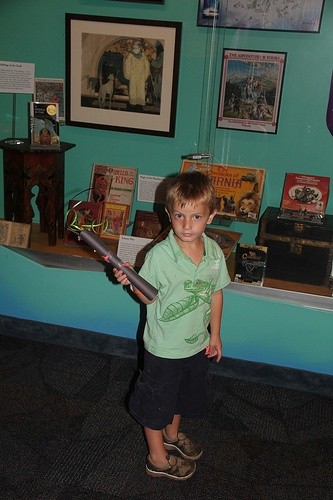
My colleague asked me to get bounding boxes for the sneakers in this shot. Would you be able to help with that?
[145,454,196,480]
[163,428,203,460]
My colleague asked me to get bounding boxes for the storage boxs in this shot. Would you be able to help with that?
[255,207,333,287]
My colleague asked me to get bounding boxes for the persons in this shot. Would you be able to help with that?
[113,171,232,479]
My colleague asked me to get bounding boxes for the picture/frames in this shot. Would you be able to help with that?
[216,48,288,134]
[64,12,183,138]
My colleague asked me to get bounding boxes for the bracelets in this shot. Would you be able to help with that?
[130,284,142,295]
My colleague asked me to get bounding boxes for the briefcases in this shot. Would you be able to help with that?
[256,206,333,288]
